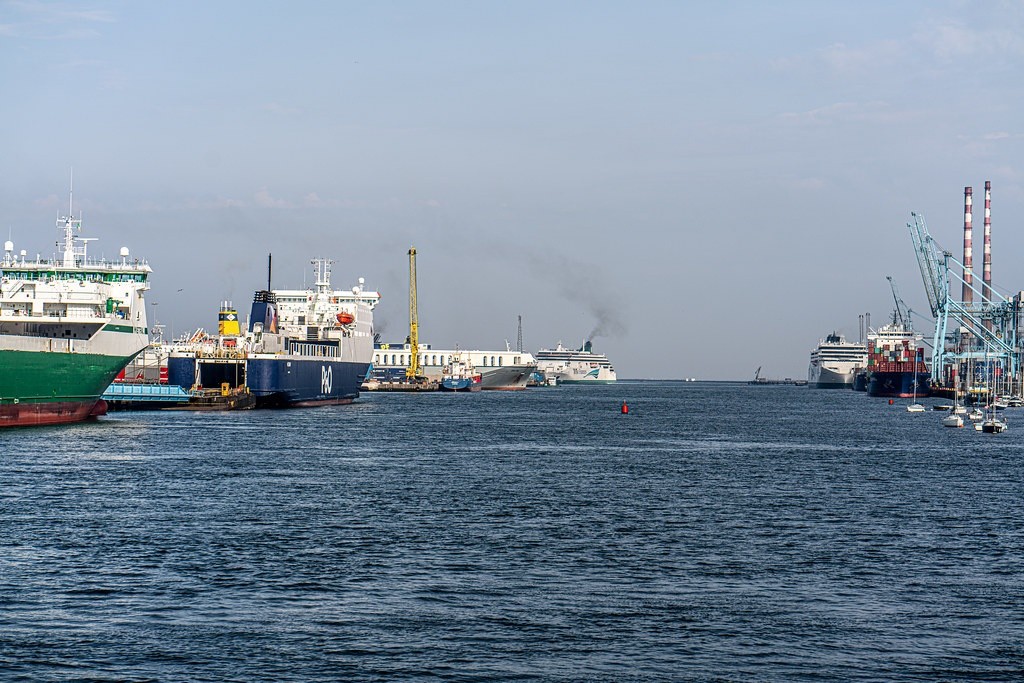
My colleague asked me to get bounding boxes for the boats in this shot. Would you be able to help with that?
[1011,363,1022,407]
[974,420,984,432]
[164,252,382,405]
[943,340,964,427]
[908,347,926,413]
[810,332,867,388]
[439,345,483,392]
[535,338,618,384]
[989,397,1009,410]
[968,405,984,420]
[1,168,152,427]
[953,355,969,414]
[933,404,956,411]
[983,366,1006,433]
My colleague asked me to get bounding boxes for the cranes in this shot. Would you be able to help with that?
[405,244,428,383]
[516,314,524,352]
